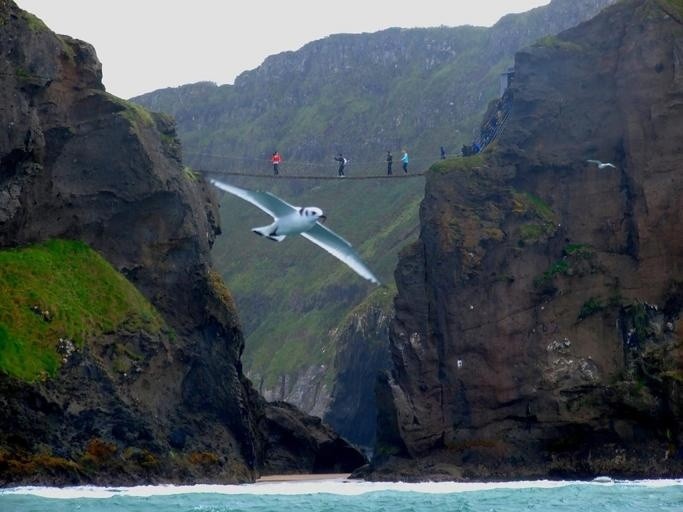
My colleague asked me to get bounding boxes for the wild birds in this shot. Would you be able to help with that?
[210,178,389,290]
[587,158,618,171]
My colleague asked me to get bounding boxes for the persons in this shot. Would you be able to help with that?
[438,145,446,160]
[399,150,408,175]
[455,143,479,157]
[384,150,392,175]
[270,151,281,176]
[334,153,346,177]
